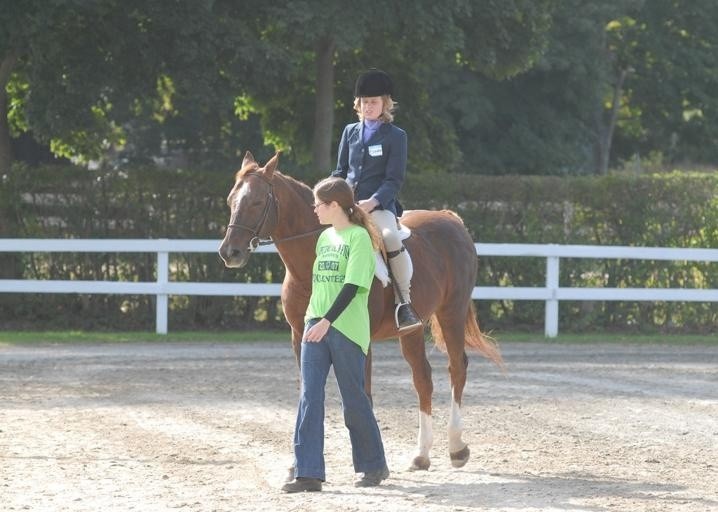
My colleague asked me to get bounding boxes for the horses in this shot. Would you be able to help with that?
[217,149,511,481]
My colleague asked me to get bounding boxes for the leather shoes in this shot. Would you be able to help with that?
[280,476,323,494]
[392,301,421,328]
[351,463,391,487]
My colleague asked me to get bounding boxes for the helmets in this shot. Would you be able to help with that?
[353,67,397,98]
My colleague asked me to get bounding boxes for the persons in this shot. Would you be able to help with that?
[330,69,422,336]
[281,177,390,493]
